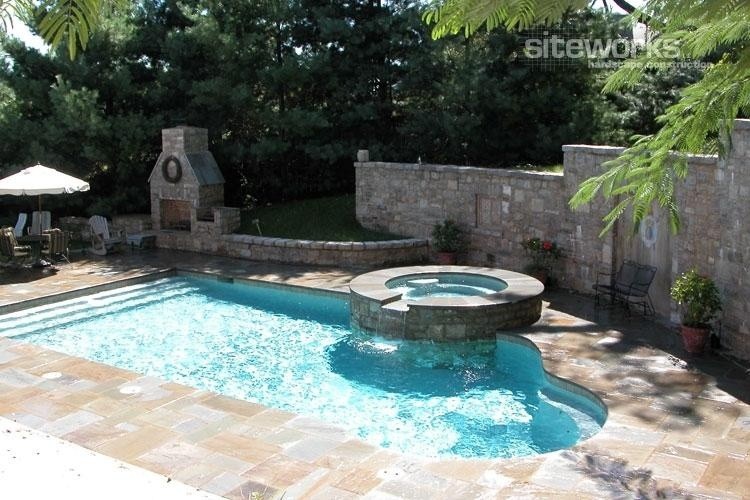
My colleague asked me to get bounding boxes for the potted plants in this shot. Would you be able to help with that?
[428,219,471,264]
[668,268,725,355]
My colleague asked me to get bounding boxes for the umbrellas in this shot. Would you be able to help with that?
[0,161,91,235]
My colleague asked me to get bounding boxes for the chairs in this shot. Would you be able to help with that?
[1,209,70,278]
[87,215,121,256]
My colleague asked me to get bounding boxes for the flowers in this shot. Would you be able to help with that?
[518,238,567,275]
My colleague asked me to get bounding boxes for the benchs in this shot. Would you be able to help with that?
[593,259,659,323]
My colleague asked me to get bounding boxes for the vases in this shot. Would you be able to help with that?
[525,269,546,284]
